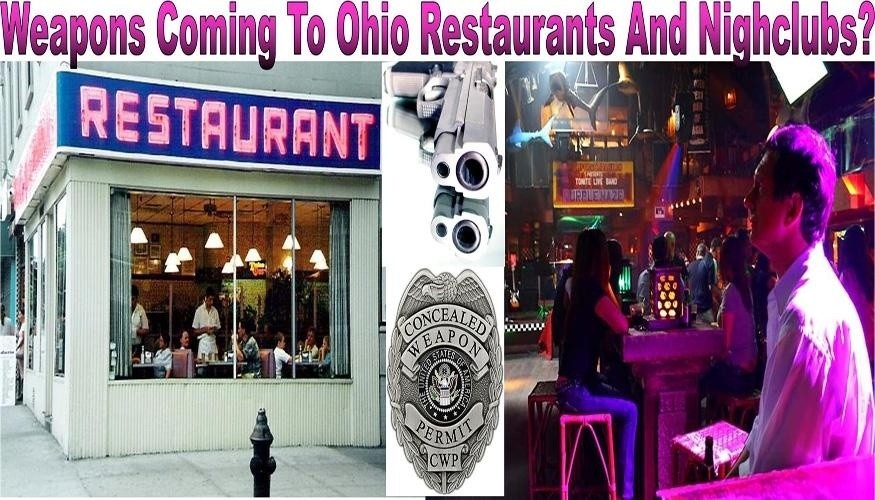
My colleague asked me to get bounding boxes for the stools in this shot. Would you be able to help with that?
[528,382,761,500]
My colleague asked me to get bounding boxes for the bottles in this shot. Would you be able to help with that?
[140,345,144,365]
[307,345,313,363]
[700,435,716,480]
[682,289,692,326]
[298,345,303,363]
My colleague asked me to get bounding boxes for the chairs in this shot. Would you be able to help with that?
[172,348,195,378]
[259,348,276,378]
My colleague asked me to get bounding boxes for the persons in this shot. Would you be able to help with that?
[127,283,331,380]
[740,121,875,483]
[0,304,14,337]
[504,246,529,318]
[547,223,873,500]
[12,308,34,404]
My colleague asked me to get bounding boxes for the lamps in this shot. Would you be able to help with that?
[131,196,329,274]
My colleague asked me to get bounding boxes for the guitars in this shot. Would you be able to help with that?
[509,264,520,308]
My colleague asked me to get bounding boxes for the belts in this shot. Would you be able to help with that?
[556,380,578,395]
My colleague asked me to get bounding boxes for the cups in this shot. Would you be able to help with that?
[632,307,644,331]
[201,353,205,362]
[223,352,228,362]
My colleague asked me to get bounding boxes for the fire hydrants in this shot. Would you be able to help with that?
[250,407,276,498]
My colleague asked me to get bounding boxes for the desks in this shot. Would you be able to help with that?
[133,363,157,378]
[621,322,724,500]
